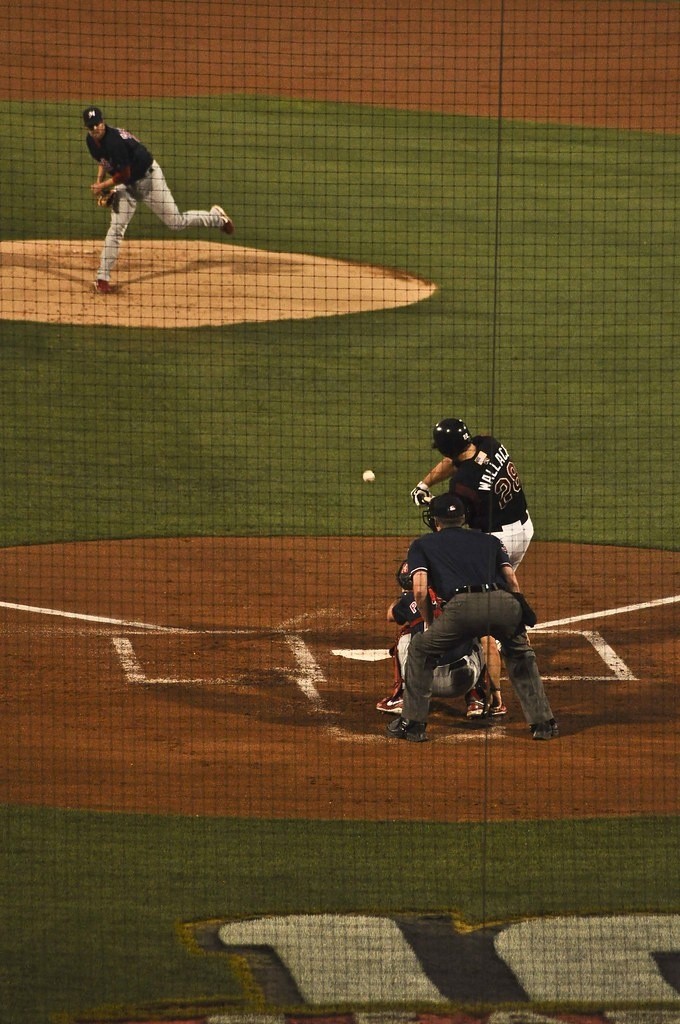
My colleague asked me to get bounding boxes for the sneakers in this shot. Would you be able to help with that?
[387,715,428,741]
[211,205,235,234]
[466,697,508,717]
[375,692,405,713]
[529,720,559,740]
[94,279,109,293]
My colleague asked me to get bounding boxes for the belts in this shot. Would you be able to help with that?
[440,582,503,608]
[481,512,528,534]
[136,168,154,181]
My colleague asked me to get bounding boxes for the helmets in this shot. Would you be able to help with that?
[432,418,472,458]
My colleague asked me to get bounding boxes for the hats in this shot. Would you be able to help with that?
[429,494,464,518]
[82,108,102,127]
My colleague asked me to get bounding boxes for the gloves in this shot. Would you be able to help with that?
[410,481,432,507]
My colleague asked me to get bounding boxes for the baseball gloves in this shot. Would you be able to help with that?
[96,189,117,208]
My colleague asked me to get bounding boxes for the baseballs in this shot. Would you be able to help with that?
[362,469,376,484]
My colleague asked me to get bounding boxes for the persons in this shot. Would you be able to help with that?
[83,107,234,293]
[375,417,557,739]
